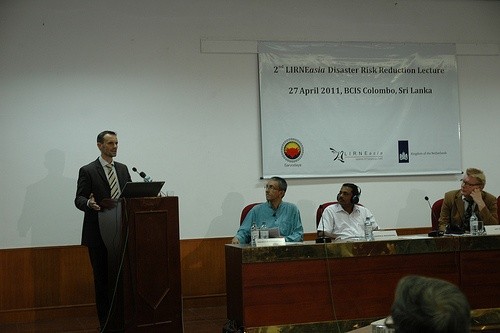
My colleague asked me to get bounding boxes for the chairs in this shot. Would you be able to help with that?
[240,198,445,233]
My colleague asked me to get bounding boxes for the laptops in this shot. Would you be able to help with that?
[118,181,165,199]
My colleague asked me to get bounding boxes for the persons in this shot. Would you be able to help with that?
[74,131,132,333]
[391,276,471,333]
[231,177,304,245]
[317,183,380,240]
[439,168,498,233]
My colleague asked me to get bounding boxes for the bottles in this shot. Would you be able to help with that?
[365,217,372,239]
[251,222,260,247]
[470,212,478,235]
[260,222,269,239]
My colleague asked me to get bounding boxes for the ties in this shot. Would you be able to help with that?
[106,163,119,200]
[463,197,474,230]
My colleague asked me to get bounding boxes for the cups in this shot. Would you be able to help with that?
[477,221,484,235]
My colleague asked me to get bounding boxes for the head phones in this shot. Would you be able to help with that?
[336,187,361,204]
[445,224,462,234]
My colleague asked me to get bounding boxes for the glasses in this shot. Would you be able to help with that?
[460,179,481,186]
[264,184,279,190]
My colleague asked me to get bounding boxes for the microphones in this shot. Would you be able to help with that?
[316,205,331,243]
[425,196,444,237]
[132,167,151,182]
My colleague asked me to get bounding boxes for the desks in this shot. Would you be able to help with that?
[224,231,500,333]
